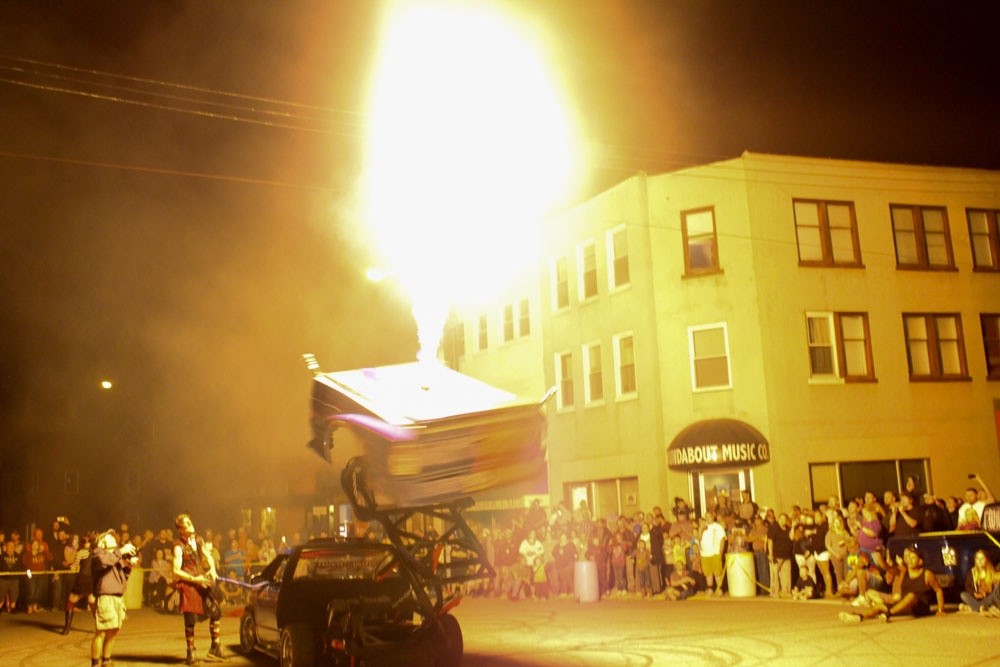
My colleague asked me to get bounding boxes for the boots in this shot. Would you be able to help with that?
[91,603,97,619]
[61,600,76,635]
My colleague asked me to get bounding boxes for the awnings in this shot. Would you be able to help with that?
[667,418,771,468]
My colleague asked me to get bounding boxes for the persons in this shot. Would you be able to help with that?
[0,469,1000,667]
[90,528,137,667]
[839,547,946,624]
[170,513,229,665]
[52,521,99,635]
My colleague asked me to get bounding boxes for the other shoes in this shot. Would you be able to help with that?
[958,603,971,611]
[663,587,681,601]
[838,611,861,625]
[852,594,866,607]
[187,648,198,665]
[877,612,891,623]
[705,588,724,597]
[207,643,234,661]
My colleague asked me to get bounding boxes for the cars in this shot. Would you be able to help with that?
[239,537,417,667]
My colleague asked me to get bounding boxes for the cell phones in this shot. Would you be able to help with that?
[969,475,976,479]
